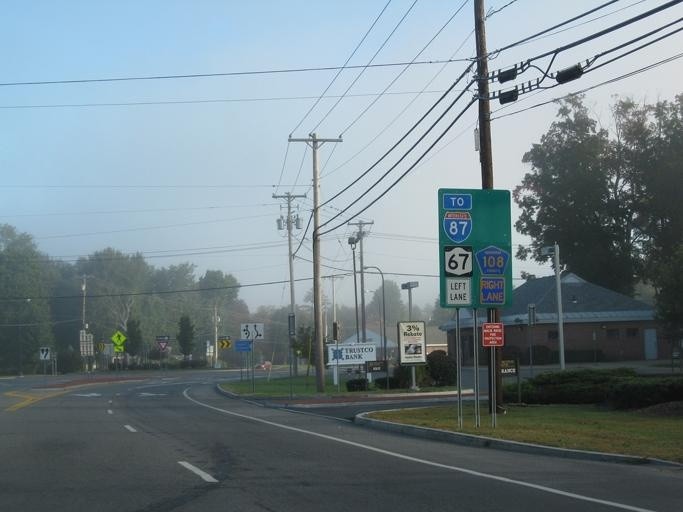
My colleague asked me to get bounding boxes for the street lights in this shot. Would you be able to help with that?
[541,241,565,369]
[79,273,88,328]
[363,265,390,391]
[401,280,420,389]
[15,298,32,379]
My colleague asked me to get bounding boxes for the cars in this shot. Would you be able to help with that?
[255,360,272,370]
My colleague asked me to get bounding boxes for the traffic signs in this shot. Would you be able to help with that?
[437,188,511,308]
